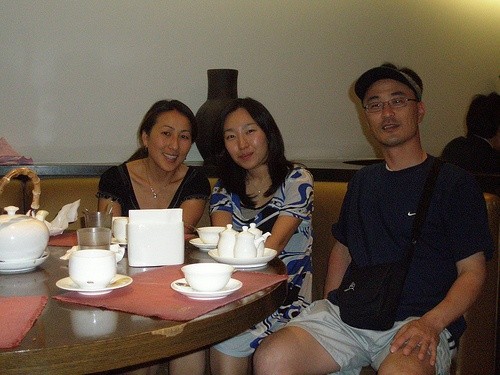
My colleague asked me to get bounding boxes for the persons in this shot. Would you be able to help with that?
[254,63,493,375]
[439,92,500,196]
[170,96,314,375]
[97,99,212,375]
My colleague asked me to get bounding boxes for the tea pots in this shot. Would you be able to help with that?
[248,223,271,257]
[233,226,265,258]
[0,168,50,262]
[217,224,239,258]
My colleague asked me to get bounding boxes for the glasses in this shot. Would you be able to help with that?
[362,97,418,113]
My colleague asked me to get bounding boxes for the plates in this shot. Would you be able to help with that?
[208,248,278,267]
[170,278,243,300]
[56,273,133,295]
[0,252,49,274]
[189,238,218,252]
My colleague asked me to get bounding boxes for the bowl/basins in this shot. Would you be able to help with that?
[196,226,227,244]
[181,263,233,292]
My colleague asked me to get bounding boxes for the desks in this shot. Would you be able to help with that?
[0,230,287,375]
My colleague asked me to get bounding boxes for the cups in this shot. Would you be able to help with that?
[85,212,112,229]
[68,249,117,289]
[77,227,111,250]
[111,217,128,243]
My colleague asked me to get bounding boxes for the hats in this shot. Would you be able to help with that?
[355,66,422,105]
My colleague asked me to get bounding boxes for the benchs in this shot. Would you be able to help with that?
[0,161,500,375]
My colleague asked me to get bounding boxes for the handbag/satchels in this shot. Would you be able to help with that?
[336,258,409,331]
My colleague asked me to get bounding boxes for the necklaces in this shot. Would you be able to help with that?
[145,161,174,200]
[247,176,269,194]
